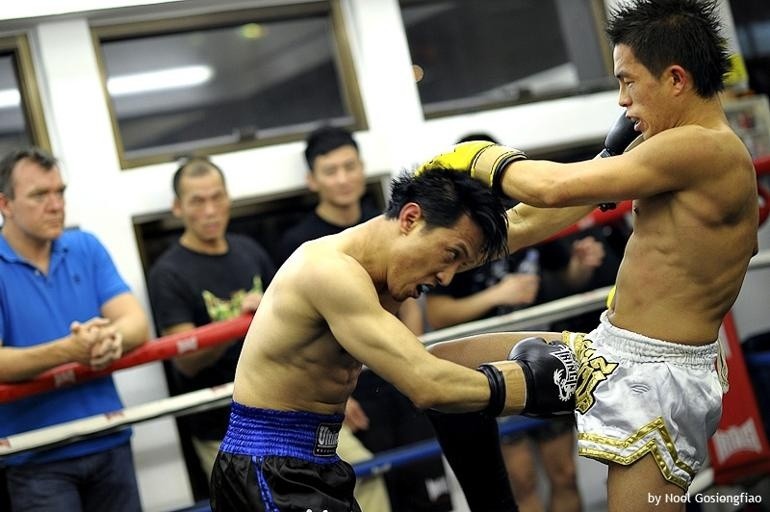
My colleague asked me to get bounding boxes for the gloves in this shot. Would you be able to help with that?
[415,139,528,188]
[591,111,643,211]
[479,337,578,417]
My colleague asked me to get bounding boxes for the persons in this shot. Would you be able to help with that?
[0,148,151,512]
[150,1,758,512]
